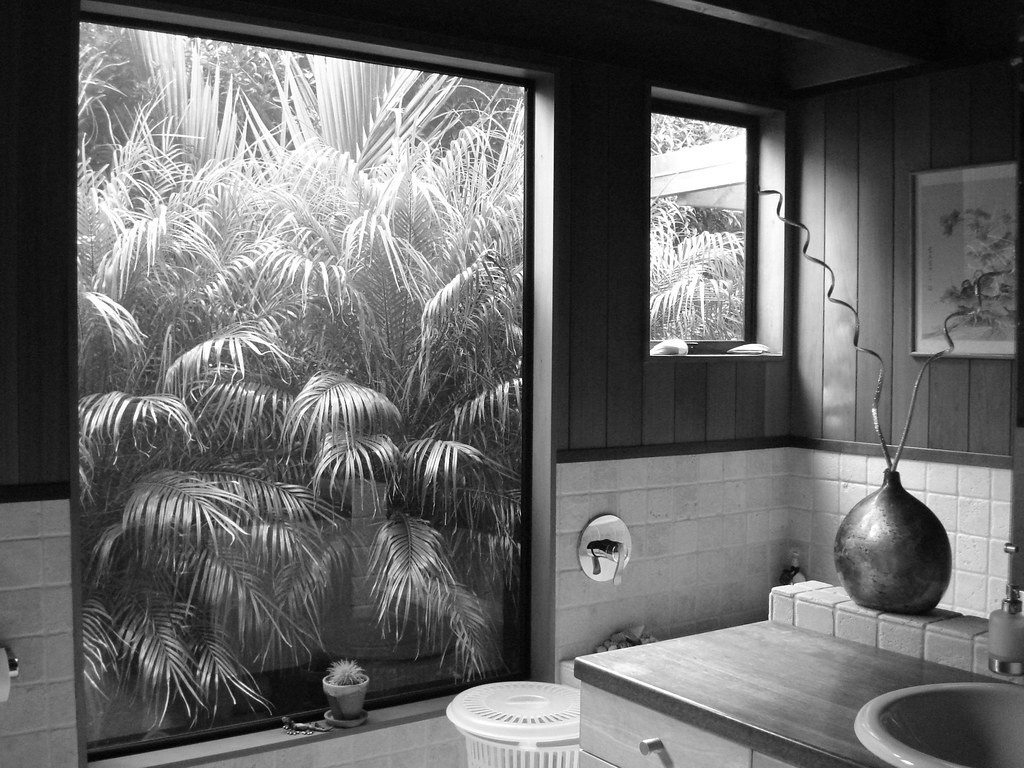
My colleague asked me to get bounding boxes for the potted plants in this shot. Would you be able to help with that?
[323,659,370,729]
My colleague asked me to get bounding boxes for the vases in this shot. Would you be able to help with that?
[833,469,951,615]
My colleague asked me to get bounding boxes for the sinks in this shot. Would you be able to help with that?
[852,681,1023,768]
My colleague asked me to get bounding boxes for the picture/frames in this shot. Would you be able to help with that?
[909,161,1021,358]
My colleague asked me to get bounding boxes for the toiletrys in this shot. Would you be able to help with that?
[988,543,1024,677]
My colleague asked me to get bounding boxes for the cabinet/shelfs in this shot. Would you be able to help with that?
[580,680,795,768]
